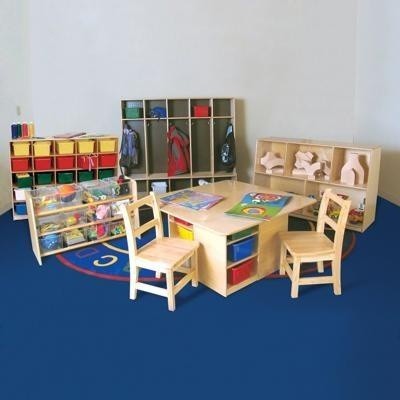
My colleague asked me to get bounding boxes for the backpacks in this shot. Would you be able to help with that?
[118,122,140,175]
[217,122,236,167]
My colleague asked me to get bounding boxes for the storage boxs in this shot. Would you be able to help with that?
[172,219,193,241]
[226,229,261,287]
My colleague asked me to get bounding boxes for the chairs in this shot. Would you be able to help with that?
[119,189,200,313]
[278,189,351,299]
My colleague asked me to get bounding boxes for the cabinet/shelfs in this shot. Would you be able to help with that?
[7,136,141,268]
[118,97,384,234]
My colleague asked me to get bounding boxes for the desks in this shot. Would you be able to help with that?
[139,178,318,300]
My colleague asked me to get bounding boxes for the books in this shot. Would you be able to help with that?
[159,189,227,211]
[223,192,292,222]
